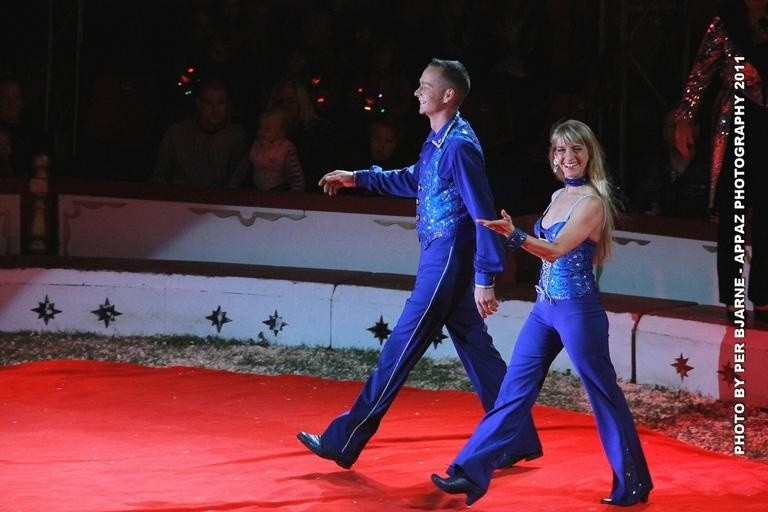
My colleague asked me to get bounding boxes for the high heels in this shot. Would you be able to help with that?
[431,472,487,506]
[600,494,648,504]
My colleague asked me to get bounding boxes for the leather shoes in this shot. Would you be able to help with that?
[754,304,768,321]
[727,305,747,320]
[492,448,543,469]
[296,432,352,469]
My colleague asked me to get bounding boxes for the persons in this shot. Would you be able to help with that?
[428,119,655,511]
[2,1,410,195]
[671,3,767,318]
[294,56,541,472]
[652,108,709,216]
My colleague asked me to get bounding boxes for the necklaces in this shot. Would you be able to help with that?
[564,177,586,187]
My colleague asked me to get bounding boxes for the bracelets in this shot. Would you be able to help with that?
[504,228,528,252]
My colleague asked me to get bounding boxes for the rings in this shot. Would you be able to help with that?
[487,304,492,309]
